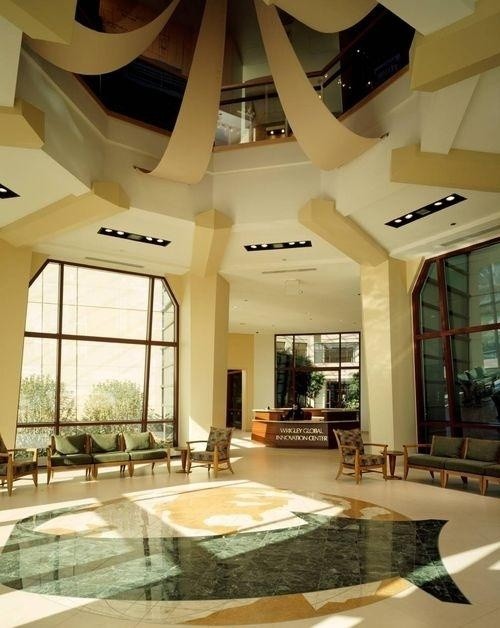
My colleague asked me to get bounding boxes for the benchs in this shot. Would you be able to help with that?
[46,432,171,485]
[402,436,500,495]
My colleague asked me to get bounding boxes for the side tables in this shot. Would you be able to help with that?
[378,449,405,481]
[175,446,194,474]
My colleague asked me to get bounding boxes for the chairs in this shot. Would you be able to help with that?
[333,428,387,485]
[185,425,235,479]
[0,433,39,496]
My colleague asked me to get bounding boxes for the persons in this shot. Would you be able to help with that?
[279,403,304,421]
